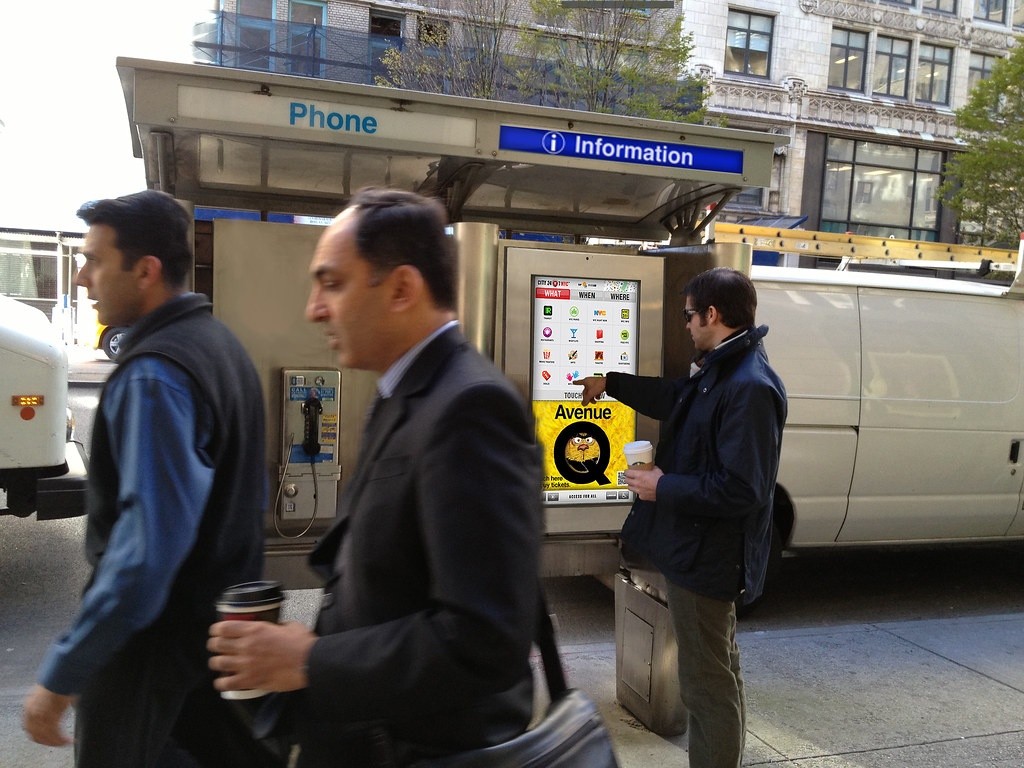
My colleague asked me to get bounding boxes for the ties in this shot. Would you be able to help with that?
[346,386,389,505]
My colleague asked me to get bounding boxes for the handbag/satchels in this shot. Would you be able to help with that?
[411,687,617,768]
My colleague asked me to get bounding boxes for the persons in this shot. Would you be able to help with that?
[573,267,787,768]
[207,187,546,768]
[14,188,269,768]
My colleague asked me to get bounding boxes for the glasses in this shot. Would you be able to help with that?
[683,308,703,322]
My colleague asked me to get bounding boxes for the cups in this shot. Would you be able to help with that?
[214,579,287,701]
[624,440,655,472]
